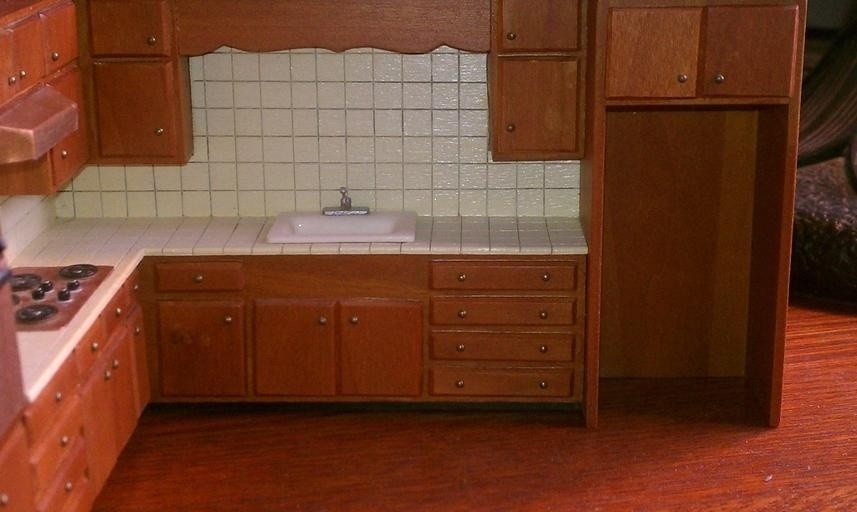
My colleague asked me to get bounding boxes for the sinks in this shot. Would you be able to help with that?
[265,212,418,243]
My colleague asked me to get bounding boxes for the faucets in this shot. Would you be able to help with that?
[340,186,351,210]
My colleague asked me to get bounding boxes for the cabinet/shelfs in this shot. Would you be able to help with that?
[252,297,427,404]
[125,254,160,421]
[2,369,30,512]
[25,371,94,511]
[487,2,587,161]
[426,256,586,403]
[597,1,808,106]
[151,256,251,404]
[78,268,138,492]
[1,1,194,196]
